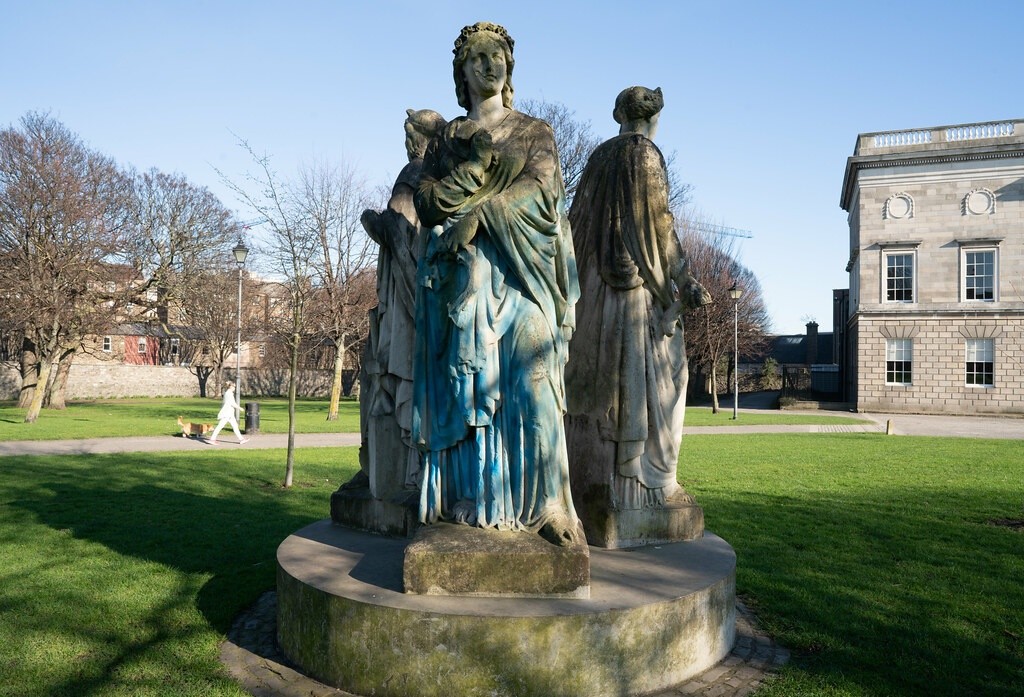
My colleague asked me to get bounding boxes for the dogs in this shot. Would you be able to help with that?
[177,415,215,438]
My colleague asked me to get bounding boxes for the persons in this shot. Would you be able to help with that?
[412,21,582,546]
[203,381,251,446]
[358,108,450,501]
[563,86,712,511]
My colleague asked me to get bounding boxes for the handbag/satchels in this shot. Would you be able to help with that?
[217,403,236,419]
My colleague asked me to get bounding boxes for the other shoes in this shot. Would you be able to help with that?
[209,440,220,445]
[240,438,251,445]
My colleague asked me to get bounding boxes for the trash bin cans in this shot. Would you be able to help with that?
[244,402,260,434]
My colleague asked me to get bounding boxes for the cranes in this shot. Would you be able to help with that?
[672,217,753,239]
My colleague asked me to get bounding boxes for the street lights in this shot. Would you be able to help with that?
[728,279,742,420]
[232,236,250,431]
